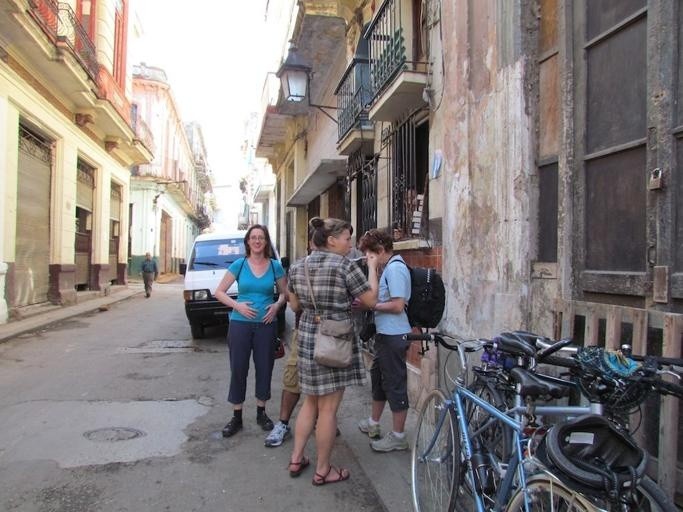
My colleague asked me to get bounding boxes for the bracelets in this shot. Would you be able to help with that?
[274,302,282,311]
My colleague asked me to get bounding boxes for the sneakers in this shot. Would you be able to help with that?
[222,416,243,437]
[369,431,409,452]
[358,418,381,437]
[256,413,274,431]
[264,420,291,447]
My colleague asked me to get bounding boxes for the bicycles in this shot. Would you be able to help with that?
[401,330,682,512]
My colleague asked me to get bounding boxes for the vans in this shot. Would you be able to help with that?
[183,231,290,340]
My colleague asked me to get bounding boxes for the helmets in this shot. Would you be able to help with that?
[536,414,648,495]
[572,346,651,414]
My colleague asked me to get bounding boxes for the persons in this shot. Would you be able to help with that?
[261,229,342,448]
[355,229,414,453]
[213,223,288,438]
[140,251,160,298]
[288,215,378,487]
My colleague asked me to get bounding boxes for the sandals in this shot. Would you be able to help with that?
[288,455,310,477]
[312,466,350,486]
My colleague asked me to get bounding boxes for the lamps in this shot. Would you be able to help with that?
[274,38,345,126]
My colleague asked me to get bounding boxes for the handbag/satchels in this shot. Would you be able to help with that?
[313,320,354,368]
[271,337,284,359]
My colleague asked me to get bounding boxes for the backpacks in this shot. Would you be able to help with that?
[385,260,445,328]
